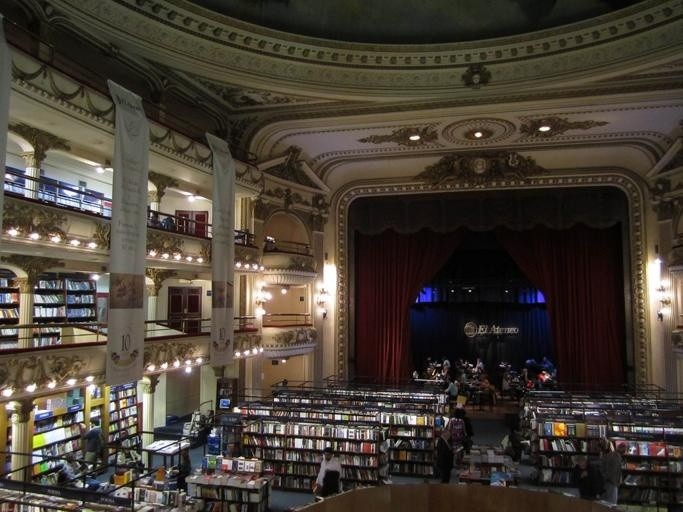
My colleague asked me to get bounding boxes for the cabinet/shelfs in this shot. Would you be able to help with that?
[141,431,273,508]
[2,166,105,217]
[1,269,99,348]
[459,389,683,512]
[0,380,141,510]
[190,381,456,494]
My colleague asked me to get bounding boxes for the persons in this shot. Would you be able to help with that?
[445,407,467,447]
[435,429,457,485]
[458,409,472,434]
[411,351,560,406]
[311,446,343,503]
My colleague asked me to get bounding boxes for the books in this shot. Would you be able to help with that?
[201,390,450,492]
[1,270,100,346]
[456,442,516,488]
[517,389,681,512]
[0,377,269,512]
